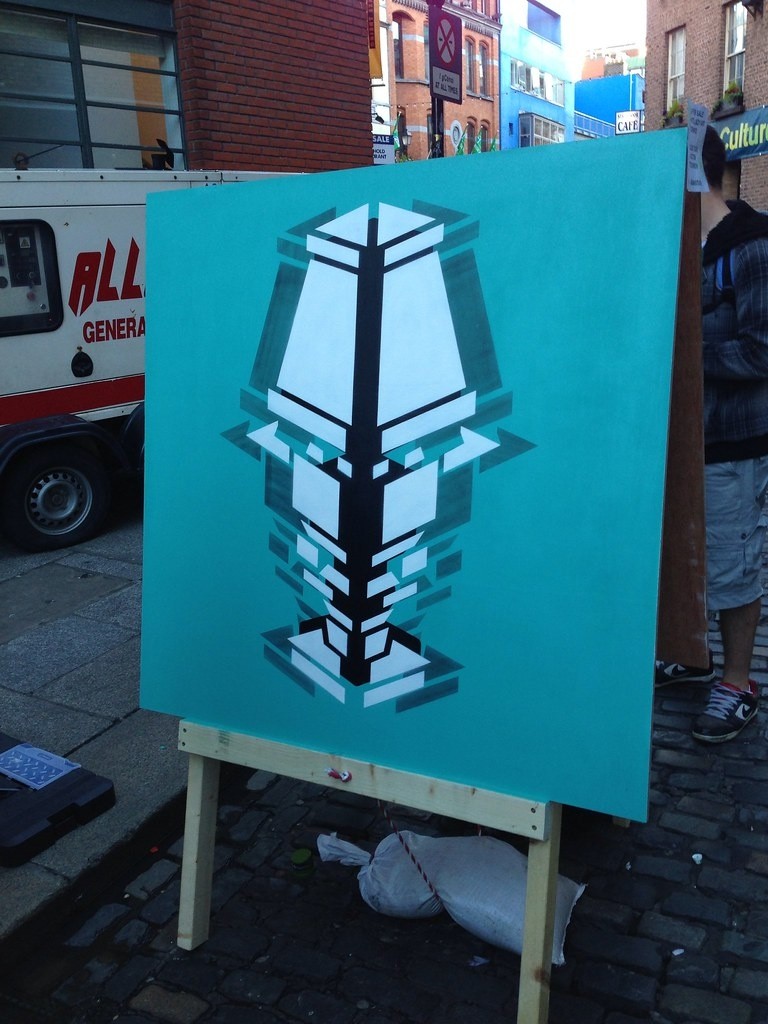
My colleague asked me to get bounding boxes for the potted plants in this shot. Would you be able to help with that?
[659,97,683,125]
[708,79,743,119]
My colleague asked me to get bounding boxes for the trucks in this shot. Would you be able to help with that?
[0,162,337,551]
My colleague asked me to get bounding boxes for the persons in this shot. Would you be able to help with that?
[653,123,768,743]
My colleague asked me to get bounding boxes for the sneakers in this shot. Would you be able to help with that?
[654,647,714,688]
[689,677,760,743]
